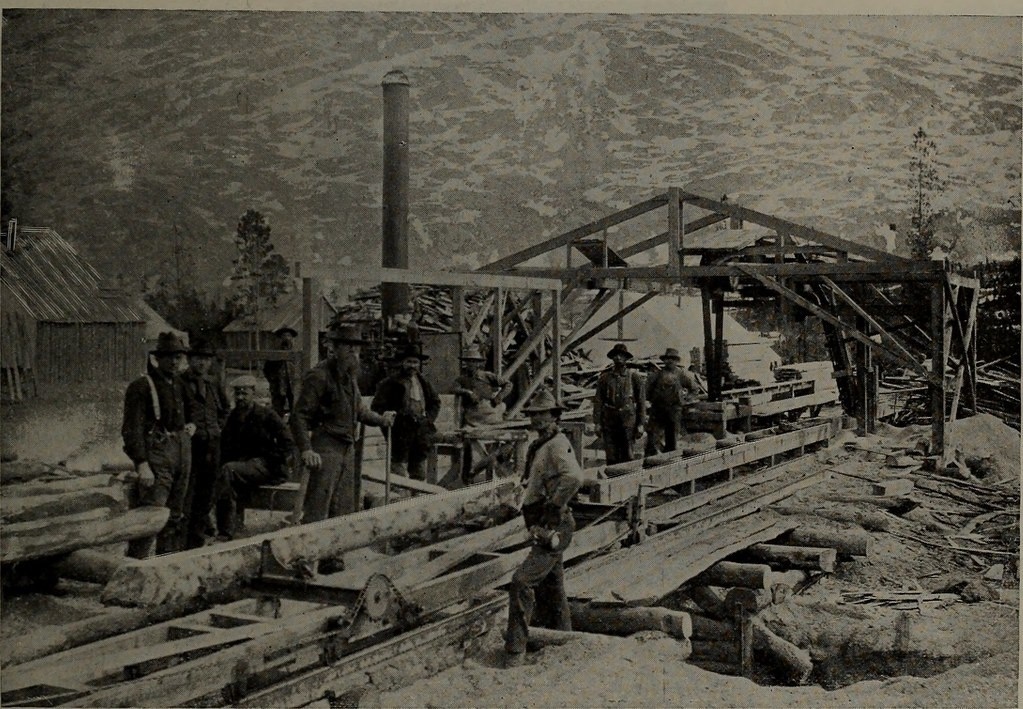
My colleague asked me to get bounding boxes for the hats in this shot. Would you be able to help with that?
[606,343,634,361]
[274,324,298,339]
[394,345,430,361]
[330,327,368,346]
[229,375,257,389]
[148,332,187,355]
[659,348,681,362]
[520,393,570,413]
[457,351,486,363]
[183,342,216,357]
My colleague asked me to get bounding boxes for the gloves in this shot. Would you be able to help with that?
[491,396,502,408]
[543,500,560,513]
[469,394,479,406]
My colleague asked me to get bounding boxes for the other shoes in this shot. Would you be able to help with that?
[318,559,346,575]
[503,648,527,671]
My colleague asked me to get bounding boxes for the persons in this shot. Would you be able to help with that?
[591,343,646,466]
[213,372,294,541]
[501,387,584,668]
[289,326,395,575]
[370,344,442,496]
[643,347,698,455]
[118,329,229,560]
[450,346,514,427]
